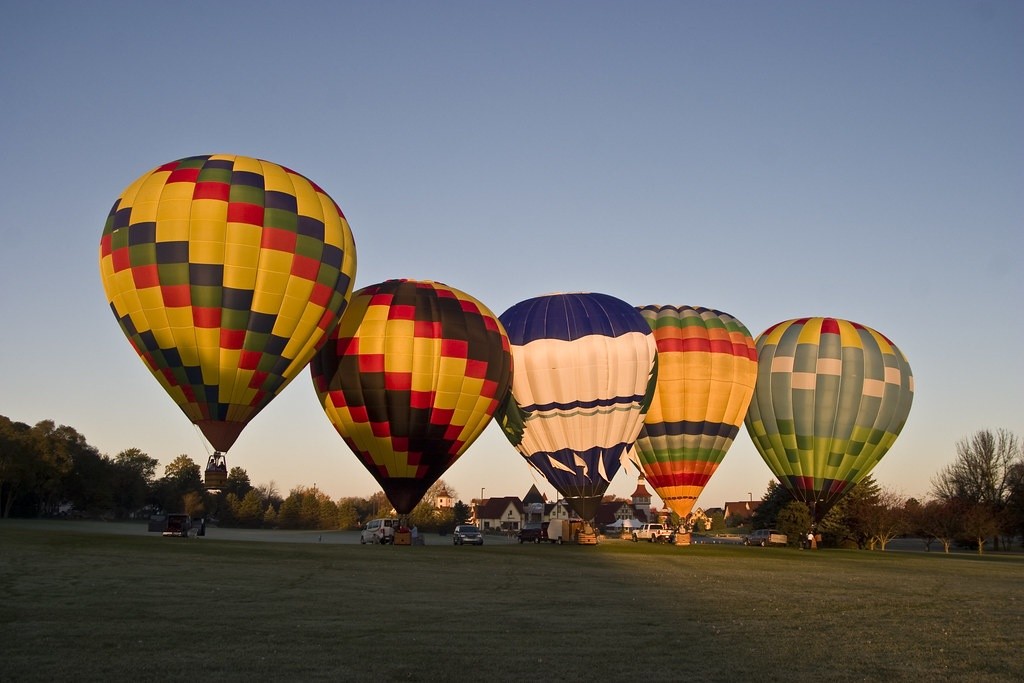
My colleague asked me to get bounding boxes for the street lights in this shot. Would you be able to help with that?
[747,492,752,501]
[481,488,485,500]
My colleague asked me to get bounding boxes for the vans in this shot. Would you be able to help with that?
[361,518,399,545]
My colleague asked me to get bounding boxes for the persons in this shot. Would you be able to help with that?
[409,523,418,546]
[574,528,581,544]
[388,522,400,545]
[209,459,218,471]
[217,462,225,472]
[798,528,823,550]
[594,527,601,543]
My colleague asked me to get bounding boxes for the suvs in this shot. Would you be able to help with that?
[517,522,549,543]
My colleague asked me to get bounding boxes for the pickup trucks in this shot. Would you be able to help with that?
[631,523,675,543]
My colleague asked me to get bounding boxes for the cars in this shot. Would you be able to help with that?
[743,529,787,547]
[453,525,483,546]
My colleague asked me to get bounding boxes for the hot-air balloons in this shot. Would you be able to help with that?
[492,292,660,545]
[625,305,760,546]
[100,154,358,490]
[310,277,513,547]
[743,318,914,549]
